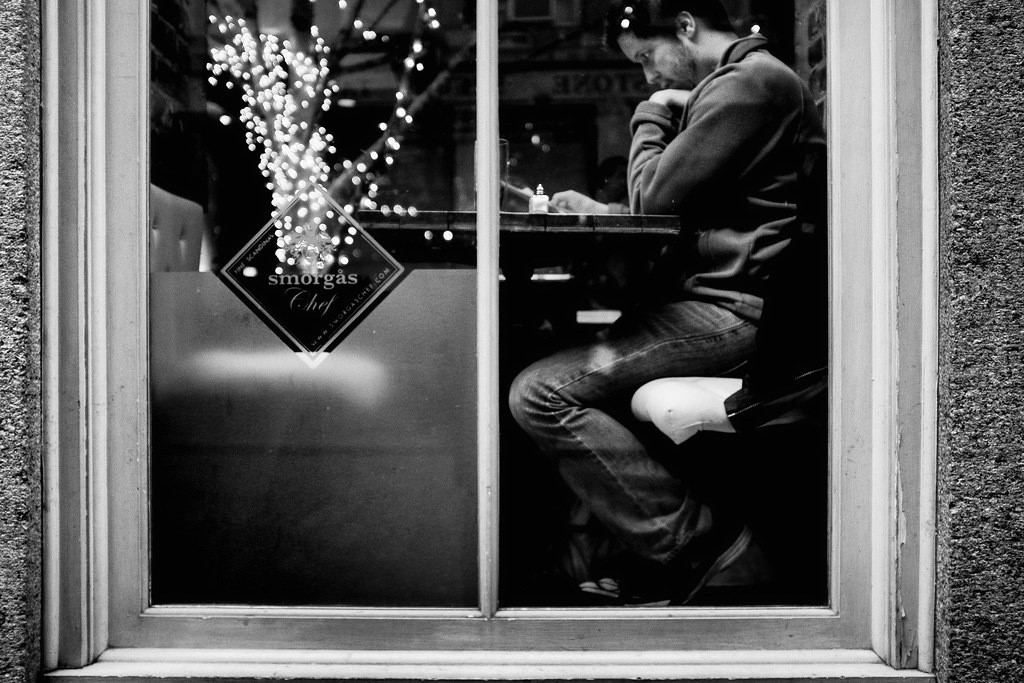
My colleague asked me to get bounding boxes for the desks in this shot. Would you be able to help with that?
[354,206,690,602]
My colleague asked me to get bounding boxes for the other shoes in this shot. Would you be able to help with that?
[669,519,751,606]
[580,573,672,607]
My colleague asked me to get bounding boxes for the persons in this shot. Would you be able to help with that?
[509,0,825,602]
